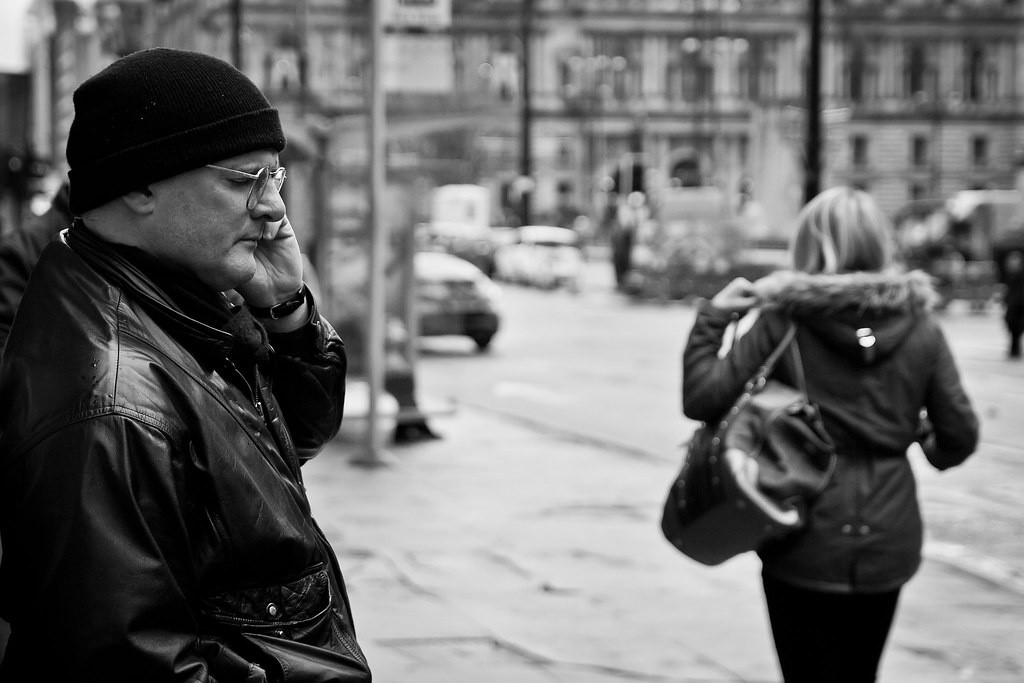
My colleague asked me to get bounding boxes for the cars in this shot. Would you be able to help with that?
[406,179,581,354]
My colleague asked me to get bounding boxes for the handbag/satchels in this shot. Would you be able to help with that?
[661,319,838,568]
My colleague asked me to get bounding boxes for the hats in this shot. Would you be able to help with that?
[62,47,286,215]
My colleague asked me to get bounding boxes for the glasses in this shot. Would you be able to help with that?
[206,165,287,209]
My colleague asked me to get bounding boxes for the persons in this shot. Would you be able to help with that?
[682,186,979,683]
[1003,249,1024,359]
[0,46,358,683]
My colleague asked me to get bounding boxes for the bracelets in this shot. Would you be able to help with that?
[243,281,307,320]
